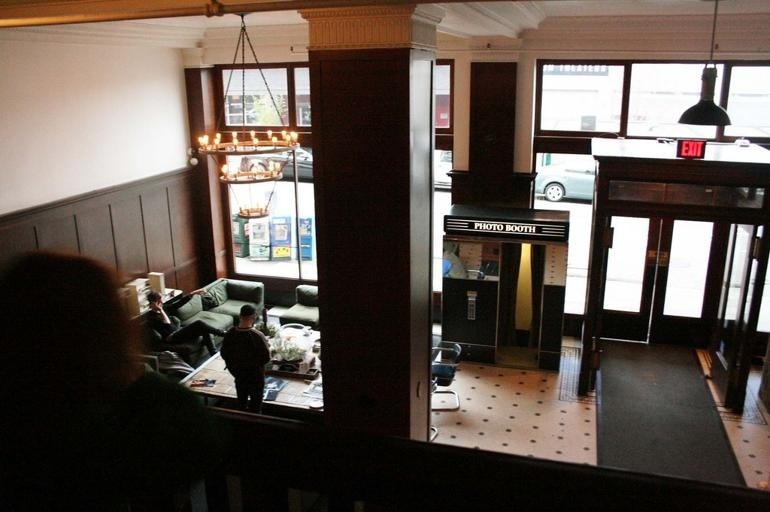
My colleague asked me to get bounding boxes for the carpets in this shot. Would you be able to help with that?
[595,339,750,485]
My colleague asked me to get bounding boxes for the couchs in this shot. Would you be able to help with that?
[146,277,266,380]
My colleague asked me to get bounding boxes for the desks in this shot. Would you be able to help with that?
[125,286,184,336]
[176,337,324,422]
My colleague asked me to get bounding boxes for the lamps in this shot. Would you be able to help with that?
[677,4,731,126]
[195,15,301,221]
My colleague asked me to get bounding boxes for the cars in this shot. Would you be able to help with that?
[536,158,596,202]
[241,147,312,180]
[434,150,453,190]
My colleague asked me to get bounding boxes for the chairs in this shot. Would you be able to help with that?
[428,338,462,412]
[280,284,321,332]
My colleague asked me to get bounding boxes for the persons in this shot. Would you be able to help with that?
[0,250,210,512]
[146,288,228,357]
[220,304,271,414]
